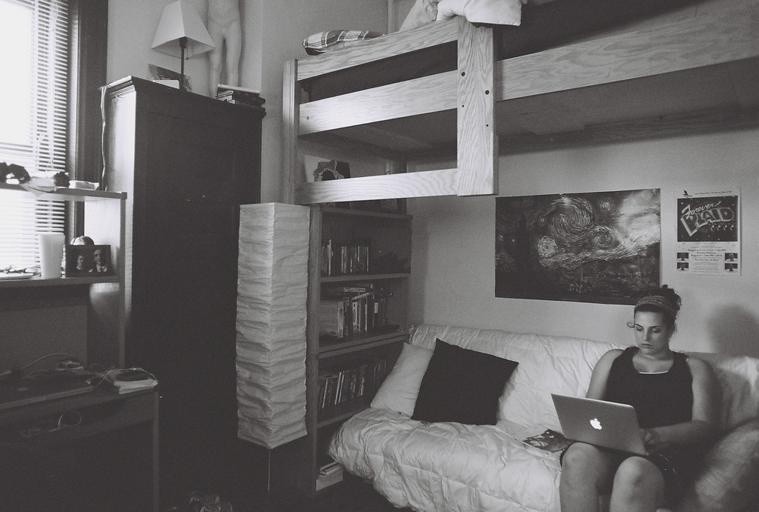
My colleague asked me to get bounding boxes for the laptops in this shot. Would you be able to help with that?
[551,392,650,457]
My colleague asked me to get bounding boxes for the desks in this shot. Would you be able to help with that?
[1,374,164,511]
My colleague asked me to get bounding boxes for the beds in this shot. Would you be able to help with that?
[279,0,758,201]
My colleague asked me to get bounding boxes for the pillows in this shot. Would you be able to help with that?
[304,31,386,54]
[370,339,433,417]
[410,337,521,428]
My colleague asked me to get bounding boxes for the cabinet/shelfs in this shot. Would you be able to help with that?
[305,203,410,458]
[108,86,263,400]
[1,179,128,290]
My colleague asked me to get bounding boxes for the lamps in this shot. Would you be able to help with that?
[232,198,309,509]
[151,0,218,96]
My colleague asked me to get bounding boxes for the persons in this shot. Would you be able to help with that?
[89,246,112,272]
[559,284,719,512]
[73,255,89,271]
[207,0,242,100]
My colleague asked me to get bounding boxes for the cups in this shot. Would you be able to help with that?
[36,232,65,279]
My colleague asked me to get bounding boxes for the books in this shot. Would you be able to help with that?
[318,237,387,477]
[92,367,158,395]
[215,90,266,112]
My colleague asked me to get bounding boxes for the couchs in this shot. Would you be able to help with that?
[324,318,759,510]
